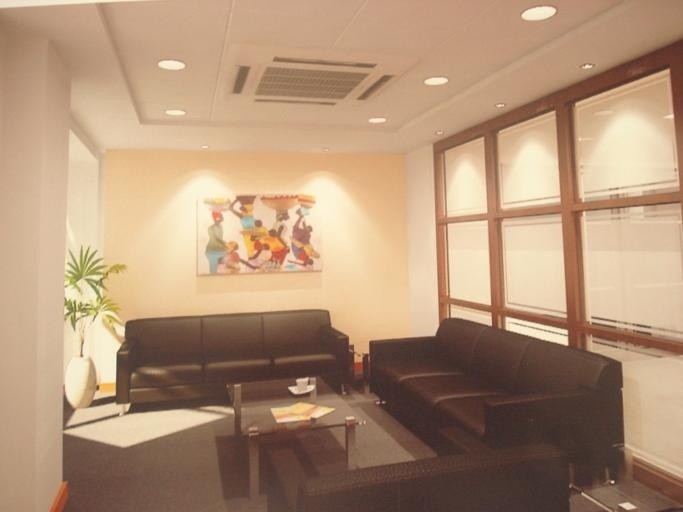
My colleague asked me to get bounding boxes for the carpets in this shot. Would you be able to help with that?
[215,430,347,499]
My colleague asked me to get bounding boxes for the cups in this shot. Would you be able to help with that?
[296,377,309,392]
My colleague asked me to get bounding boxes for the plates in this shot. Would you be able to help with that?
[288,384,316,396]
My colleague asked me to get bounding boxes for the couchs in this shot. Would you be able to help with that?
[366,317,626,487]
[115,308,351,413]
[251,434,574,512]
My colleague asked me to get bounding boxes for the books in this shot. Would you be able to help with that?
[268,406,311,424]
[283,401,336,420]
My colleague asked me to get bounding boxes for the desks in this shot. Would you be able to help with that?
[223,372,418,486]
[341,360,374,396]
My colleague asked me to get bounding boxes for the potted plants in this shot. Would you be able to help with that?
[62,244,128,410]
